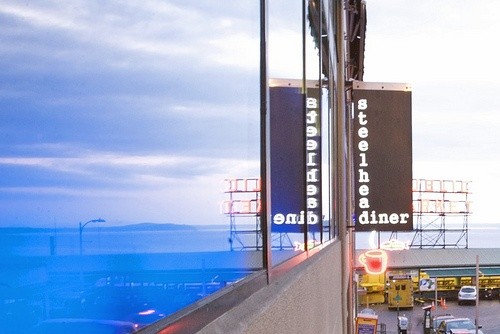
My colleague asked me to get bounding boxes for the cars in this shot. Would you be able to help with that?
[433,313,455,331]
[437,318,483,334]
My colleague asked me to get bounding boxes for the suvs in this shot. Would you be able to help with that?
[458,286,478,304]
